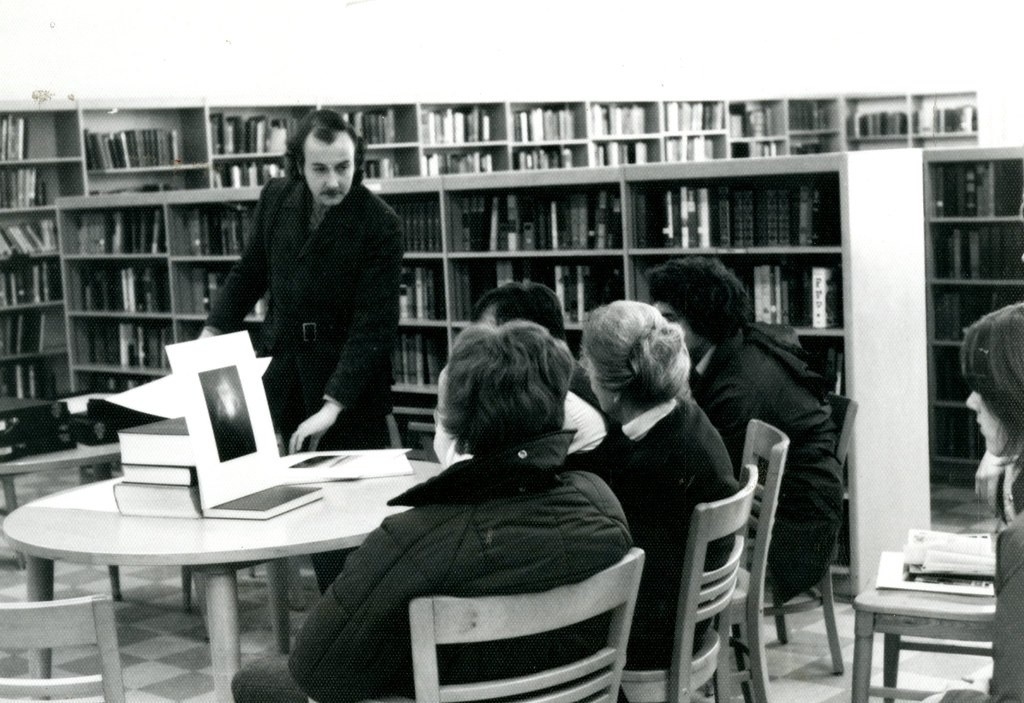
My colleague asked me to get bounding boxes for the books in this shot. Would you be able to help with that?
[112,330,413,520]
[77,320,170,371]
[83,126,178,168]
[0,115,60,399]
[423,102,975,176]
[210,111,401,190]
[875,529,997,596]
[931,161,1024,458]
[72,266,158,314]
[86,374,141,395]
[76,209,161,254]
[204,182,844,397]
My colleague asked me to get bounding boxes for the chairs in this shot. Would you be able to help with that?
[729,414,794,703]
[847,563,1004,703]
[616,461,760,703]
[0,587,126,703]
[404,541,652,703]
[765,389,861,679]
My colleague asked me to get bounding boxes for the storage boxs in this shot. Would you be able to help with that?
[59,391,136,451]
[0,399,77,462]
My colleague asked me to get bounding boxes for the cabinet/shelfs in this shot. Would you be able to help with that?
[0,89,1024,596]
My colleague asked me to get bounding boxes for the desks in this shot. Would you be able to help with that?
[0,442,123,512]
[1,456,449,703]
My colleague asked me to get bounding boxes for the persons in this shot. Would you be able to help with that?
[922,300,1024,703]
[232,262,845,703]
[201,109,402,596]
